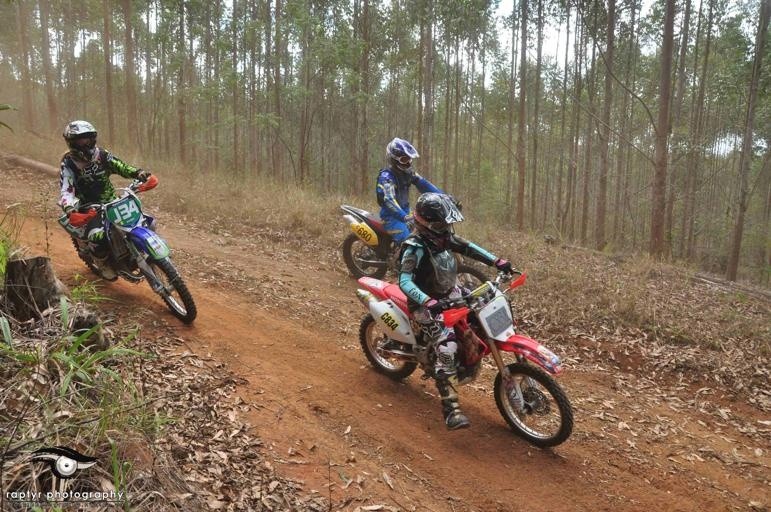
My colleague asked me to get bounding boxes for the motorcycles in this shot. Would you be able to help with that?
[57,172,198,323]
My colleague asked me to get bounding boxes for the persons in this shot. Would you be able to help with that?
[59,119,152,282]
[376,137,464,248]
[397,192,512,431]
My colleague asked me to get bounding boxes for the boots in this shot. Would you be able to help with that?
[437,371,468,430]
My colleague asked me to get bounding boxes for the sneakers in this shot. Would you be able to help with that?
[101,266,118,281]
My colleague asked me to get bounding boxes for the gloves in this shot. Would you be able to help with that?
[425,299,441,313]
[495,258,511,274]
[132,169,152,183]
[454,200,463,209]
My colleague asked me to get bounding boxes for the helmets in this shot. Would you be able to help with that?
[62,121,98,162]
[386,136,420,173]
[413,192,465,249]
